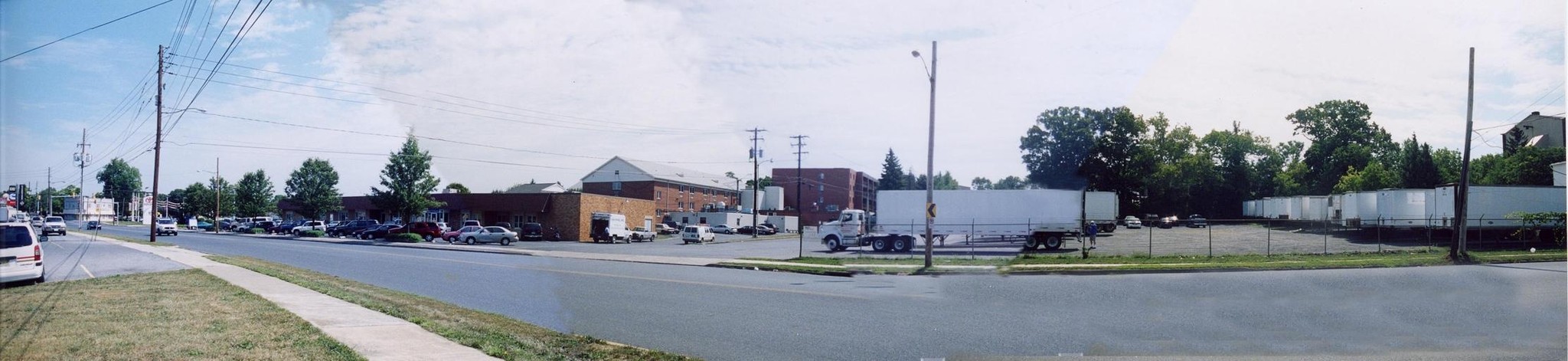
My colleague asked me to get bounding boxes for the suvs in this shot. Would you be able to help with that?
[389,222,442,242]
[520,222,543,241]
[438,223,452,235]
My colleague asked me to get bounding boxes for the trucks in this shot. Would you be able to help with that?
[589,212,632,243]
[818,186,1086,251]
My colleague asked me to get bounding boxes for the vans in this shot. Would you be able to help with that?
[462,220,480,227]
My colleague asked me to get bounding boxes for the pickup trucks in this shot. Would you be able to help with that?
[632,226,658,242]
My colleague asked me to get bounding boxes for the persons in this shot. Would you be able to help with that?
[591,225,599,243]
[1085,220,1097,249]
[603,225,610,244]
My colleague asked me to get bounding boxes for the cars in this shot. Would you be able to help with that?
[442,225,490,243]
[656,221,779,244]
[87,220,101,230]
[187,217,403,240]
[457,226,519,246]
[1124,214,1207,228]
[156,218,177,236]
[0,205,66,283]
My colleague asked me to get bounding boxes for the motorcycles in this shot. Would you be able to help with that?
[550,227,561,241]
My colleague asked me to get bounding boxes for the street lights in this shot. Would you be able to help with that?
[150,108,206,241]
[912,40,936,266]
[757,158,774,234]
[48,181,66,212]
[197,158,219,233]
